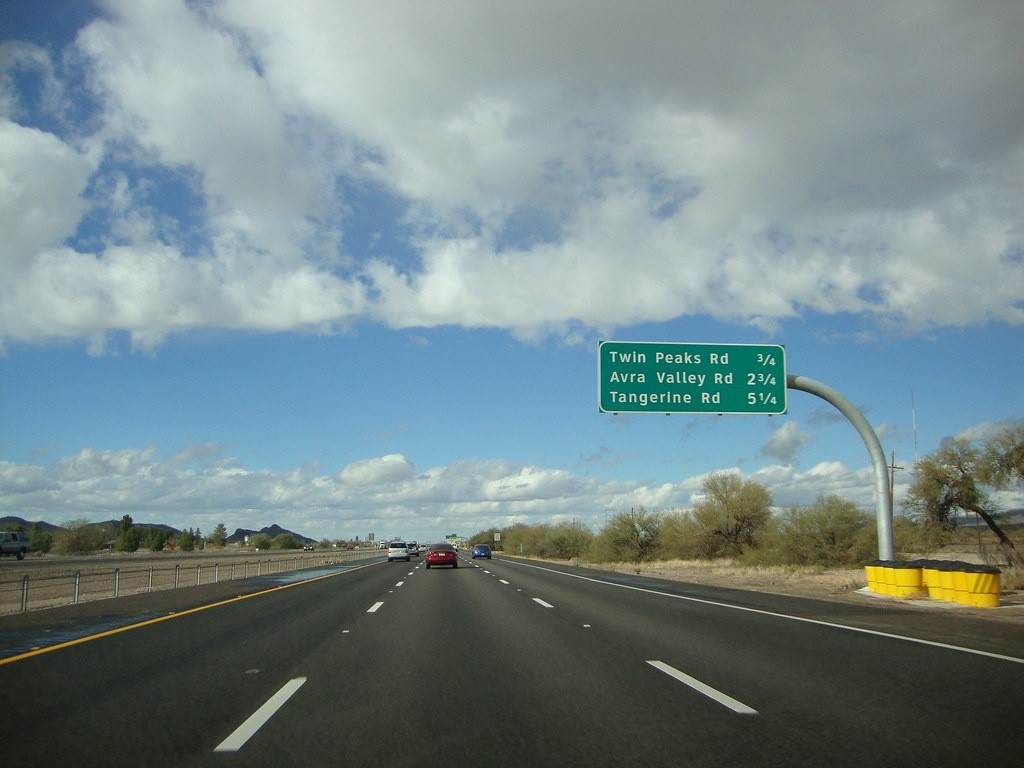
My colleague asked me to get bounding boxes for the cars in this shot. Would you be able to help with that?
[471,544,492,560]
[303,543,315,552]
[425,543,459,568]
[418,544,428,551]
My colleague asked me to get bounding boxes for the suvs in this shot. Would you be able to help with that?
[347,544,353,550]
[386,540,411,563]
[405,539,420,557]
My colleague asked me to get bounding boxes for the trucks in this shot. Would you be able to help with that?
[378,542,386,549]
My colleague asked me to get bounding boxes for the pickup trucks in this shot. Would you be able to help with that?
[0,531,32,561]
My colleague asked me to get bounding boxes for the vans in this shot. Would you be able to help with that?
[451,543,457,550]
